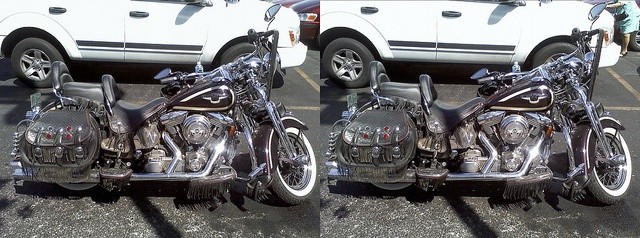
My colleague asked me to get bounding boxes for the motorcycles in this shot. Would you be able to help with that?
[5,3,319,206]
[325,0,634,205]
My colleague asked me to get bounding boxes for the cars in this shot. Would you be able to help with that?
[288,0,320,49]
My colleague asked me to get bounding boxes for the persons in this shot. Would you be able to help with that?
[604,0,640,58]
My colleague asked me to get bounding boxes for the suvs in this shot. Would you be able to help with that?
[320,1,623,88]
[0,0,308,89]
